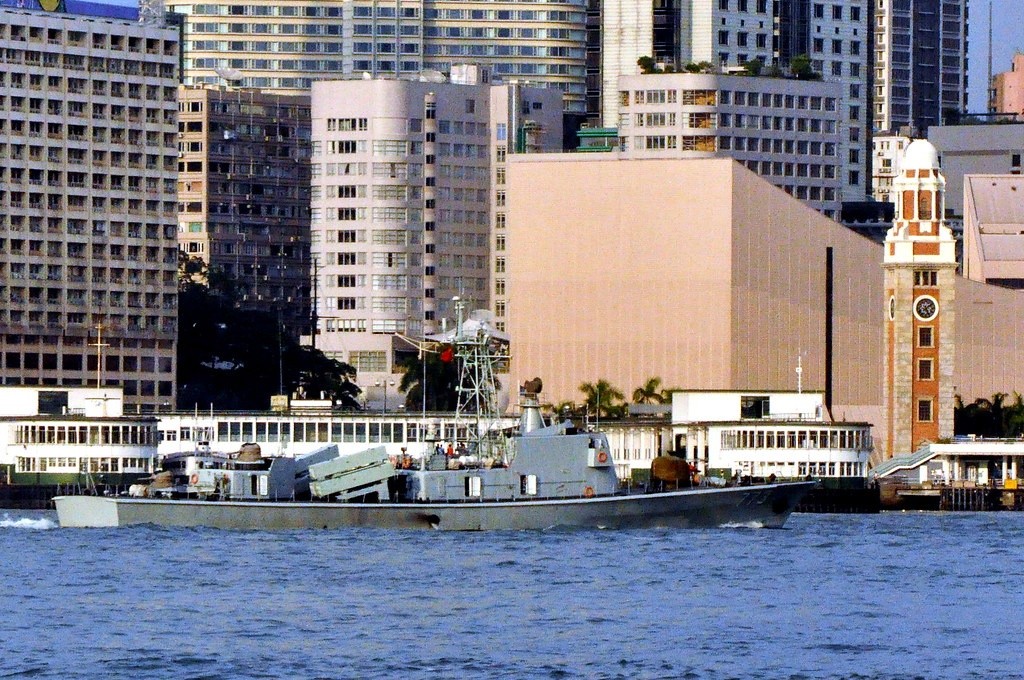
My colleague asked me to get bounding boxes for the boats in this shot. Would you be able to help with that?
[47,372,824,537]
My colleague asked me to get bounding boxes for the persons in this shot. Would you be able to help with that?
[991,473,996,487]
[719,469,724,477]
[435,442,466,456]
[734,470,739,486]
[1006,472,1010,479]
[948,471,955,487]
[941,470,946,485]
[688,460,699,483]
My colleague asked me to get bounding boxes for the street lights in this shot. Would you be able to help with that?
[375,375,396,415]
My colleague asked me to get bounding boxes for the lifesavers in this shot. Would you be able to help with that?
[584,486,594,497]
[598,452,607,462]
[191,474,198,484]
